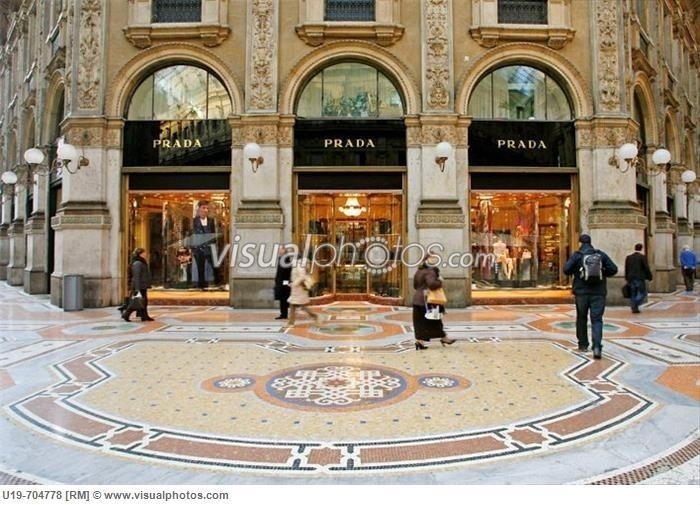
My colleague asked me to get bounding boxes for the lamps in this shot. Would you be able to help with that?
[608,143,638,172]
[663,170,697,187]
[24,148,64,175]
[338,196,367,216]
[435,141,452,172]
[1,171,37,185]
[244,143,264,173]
[56,144,89,174]
[637,149,671,176]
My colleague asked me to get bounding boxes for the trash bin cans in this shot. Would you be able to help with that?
[63,275,84,311]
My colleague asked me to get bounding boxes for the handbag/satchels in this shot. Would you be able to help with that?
[129,290,145,311]
[622,283,630,299]
[302,270,321,289]
[421,315,442,340]
[427,287,448,305]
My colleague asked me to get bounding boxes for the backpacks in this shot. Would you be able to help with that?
[574,249,604,282]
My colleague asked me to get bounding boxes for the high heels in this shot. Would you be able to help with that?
[440,340,456,347]
[415,341,428,350]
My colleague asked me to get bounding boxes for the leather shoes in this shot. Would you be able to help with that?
[141,317,154,321]
[121,315,131,321]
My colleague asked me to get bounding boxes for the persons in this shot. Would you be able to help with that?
[411,249,458,349]
[192,200,226,290]
[624,242,653,313]
[679,244,697,293]
[493,240,513,280]
[120,247,154,322]
[273,247,291,321]
[563,233,618,359]
[285,255,319,327]
[317,217,329,235]
[117,246,143,317]
